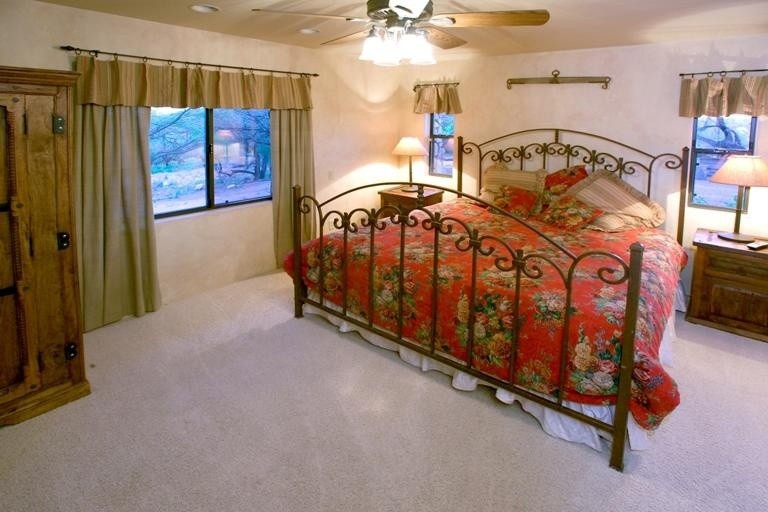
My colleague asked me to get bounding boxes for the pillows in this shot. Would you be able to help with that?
[479,161,666,235]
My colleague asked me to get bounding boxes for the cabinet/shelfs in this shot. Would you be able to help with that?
[0,64,91,430]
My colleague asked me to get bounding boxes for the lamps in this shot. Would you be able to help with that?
[359,1,436,69]
[708,154,768,243]
[392,136,429,192]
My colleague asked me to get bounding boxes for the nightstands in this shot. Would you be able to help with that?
[376,186,445,216]
[683,228,768,343]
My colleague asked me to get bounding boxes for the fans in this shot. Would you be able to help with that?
[248,1,550,50]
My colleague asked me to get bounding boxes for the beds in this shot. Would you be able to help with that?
[289,126,689,473]
